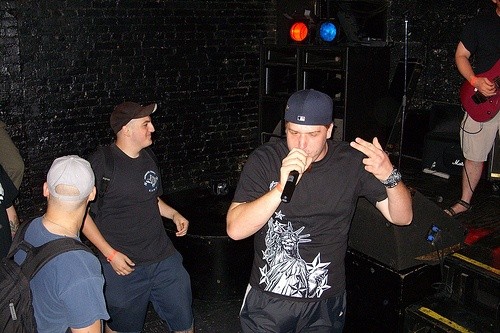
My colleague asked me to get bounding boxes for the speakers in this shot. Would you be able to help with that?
[347,181,465,271]
[257,0,390,146]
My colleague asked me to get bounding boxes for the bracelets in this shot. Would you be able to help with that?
[380,165,402,189]
[107,249,117,262]
[226,89,413,333]
[275,184,283,193]
[470,76,478,86]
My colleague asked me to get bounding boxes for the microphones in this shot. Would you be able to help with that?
[281,169,299,203]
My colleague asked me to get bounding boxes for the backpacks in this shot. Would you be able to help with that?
[0,217,95,333]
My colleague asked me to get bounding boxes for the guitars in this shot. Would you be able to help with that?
[461,59,500,123]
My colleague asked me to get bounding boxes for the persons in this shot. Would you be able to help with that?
[0,120,26,260]
[443,0,500,216]
[81,100,194,333]
[13,155,111,333]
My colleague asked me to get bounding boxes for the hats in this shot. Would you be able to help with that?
[285,88,333,125]
[110,101,157,135]
[47,155,95,201]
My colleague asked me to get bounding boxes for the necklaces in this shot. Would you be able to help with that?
[43,214,78,238]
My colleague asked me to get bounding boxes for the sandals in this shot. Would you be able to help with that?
[442,200,472,219]
